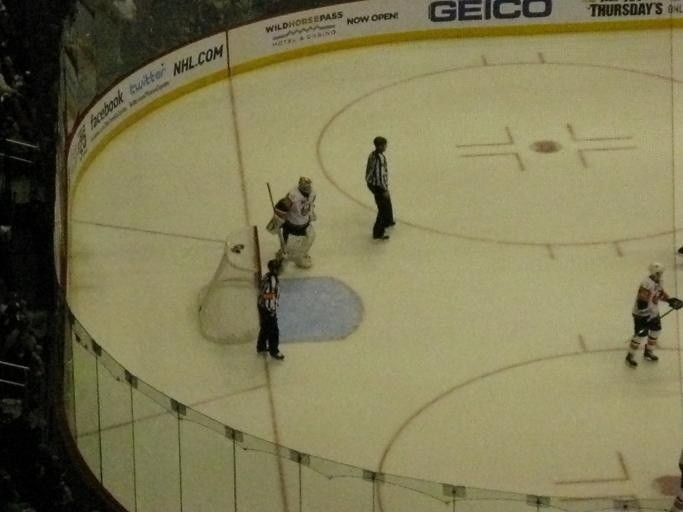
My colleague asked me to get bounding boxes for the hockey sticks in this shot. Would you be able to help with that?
[623,306,676,356]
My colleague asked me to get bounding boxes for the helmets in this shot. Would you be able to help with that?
[373,137,386,146]
[299,177,313,194]
[267,259,280,274]
[648,262,664,275]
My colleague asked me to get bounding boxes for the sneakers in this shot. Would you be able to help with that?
[273,351,284,359]
[626,352,638,366]
[264,345,268,351]
[644,349,657,360]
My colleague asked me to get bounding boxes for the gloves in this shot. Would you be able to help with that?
[635,324,648,336]
[669,298,682,309]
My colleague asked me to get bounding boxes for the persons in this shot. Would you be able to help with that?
[673,450,683,510]
[678,246,683,254]
[625,261,683,366]
[257,254,285,359]
[267,177,316,270]
[366,136,397,241]
